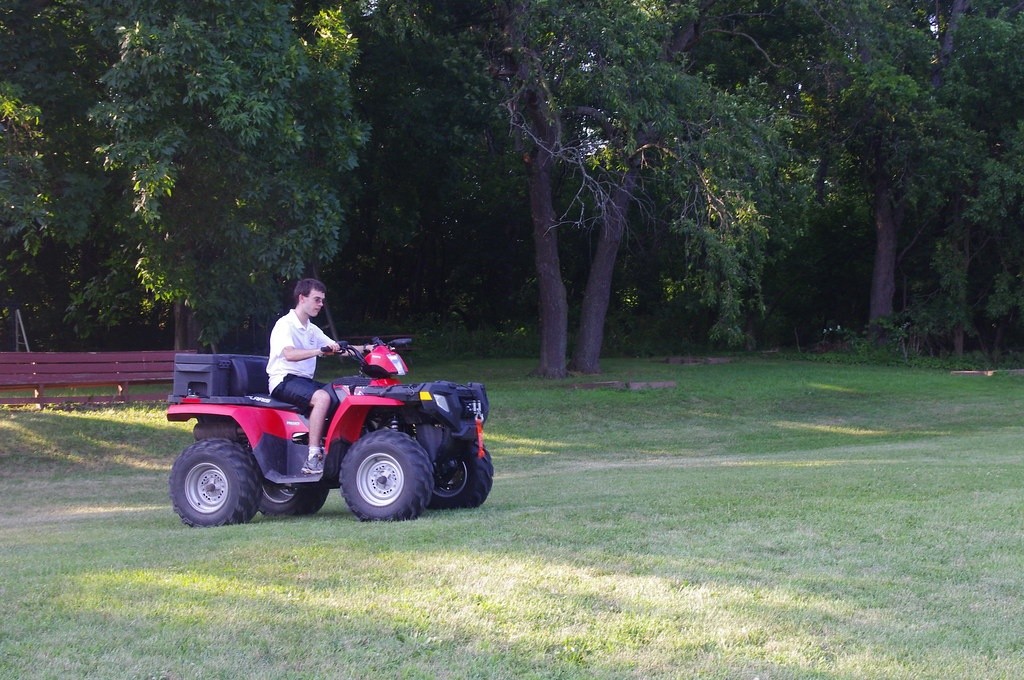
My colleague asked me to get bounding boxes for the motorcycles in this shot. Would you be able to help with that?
[164,337,494,527]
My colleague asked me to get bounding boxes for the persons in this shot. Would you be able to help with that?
[264,279,395,475]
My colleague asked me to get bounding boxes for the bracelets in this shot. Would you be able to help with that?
[363,343,369,351]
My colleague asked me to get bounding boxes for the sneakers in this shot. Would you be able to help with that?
[301,453,324,473]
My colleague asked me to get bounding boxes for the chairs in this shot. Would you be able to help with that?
[234,356,301,412]
